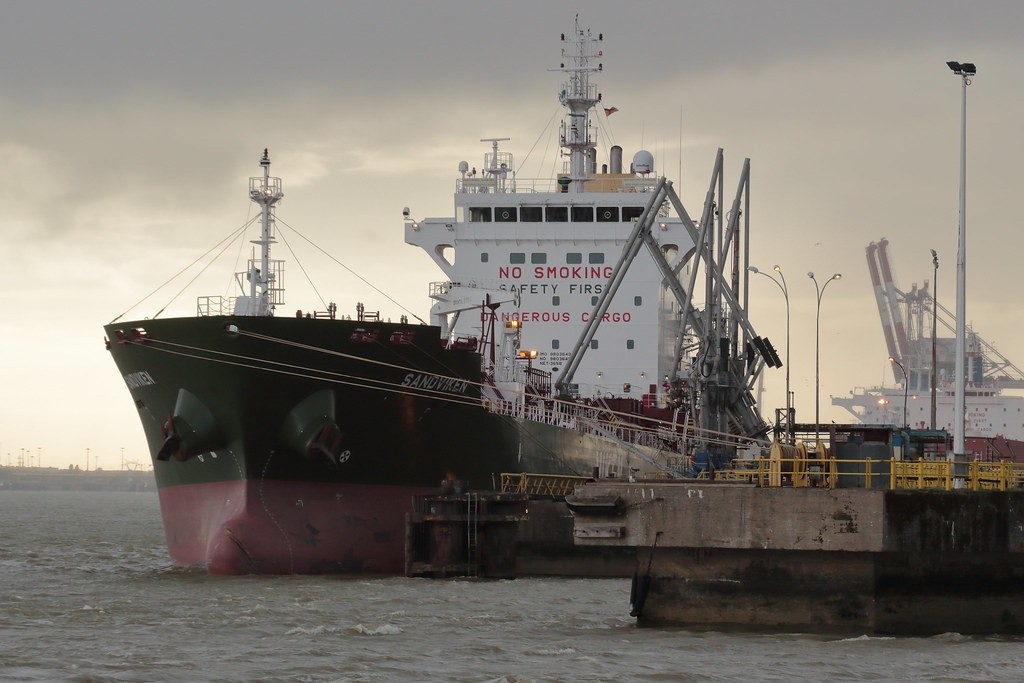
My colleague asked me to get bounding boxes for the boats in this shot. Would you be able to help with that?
[827,237,1024,467]
[101,8,805,578]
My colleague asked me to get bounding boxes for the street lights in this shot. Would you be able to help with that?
[930,247,938,430]
[746,264,790,447]
[807,271,842,445]
[945,60,977,490]
[889,356,908,432]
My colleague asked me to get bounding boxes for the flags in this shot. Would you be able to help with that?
[604,107,618,117]
